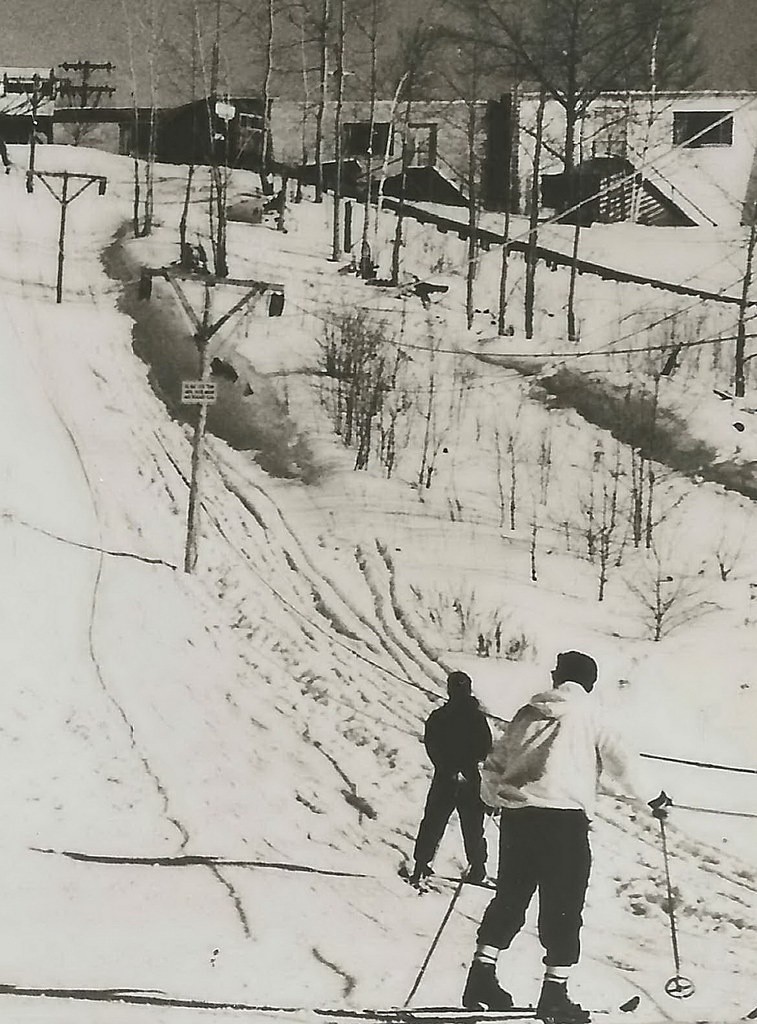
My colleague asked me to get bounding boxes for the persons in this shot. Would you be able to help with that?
[464,650,673,1024]
[409,671,497,893]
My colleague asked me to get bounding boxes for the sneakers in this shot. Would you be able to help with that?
[536,979,591,1024]
[461,957,513,1009]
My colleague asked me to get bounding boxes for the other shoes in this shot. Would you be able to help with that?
[468,871,499,889]
[414,862,434,878]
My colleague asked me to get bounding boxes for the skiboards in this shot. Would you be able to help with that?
[362,996,642,1024]
[398,865,500,893]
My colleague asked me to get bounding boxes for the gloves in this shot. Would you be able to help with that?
[649,793,673,811]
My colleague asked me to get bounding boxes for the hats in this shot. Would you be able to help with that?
[551,651,599,693]
[445,672,472,696]
